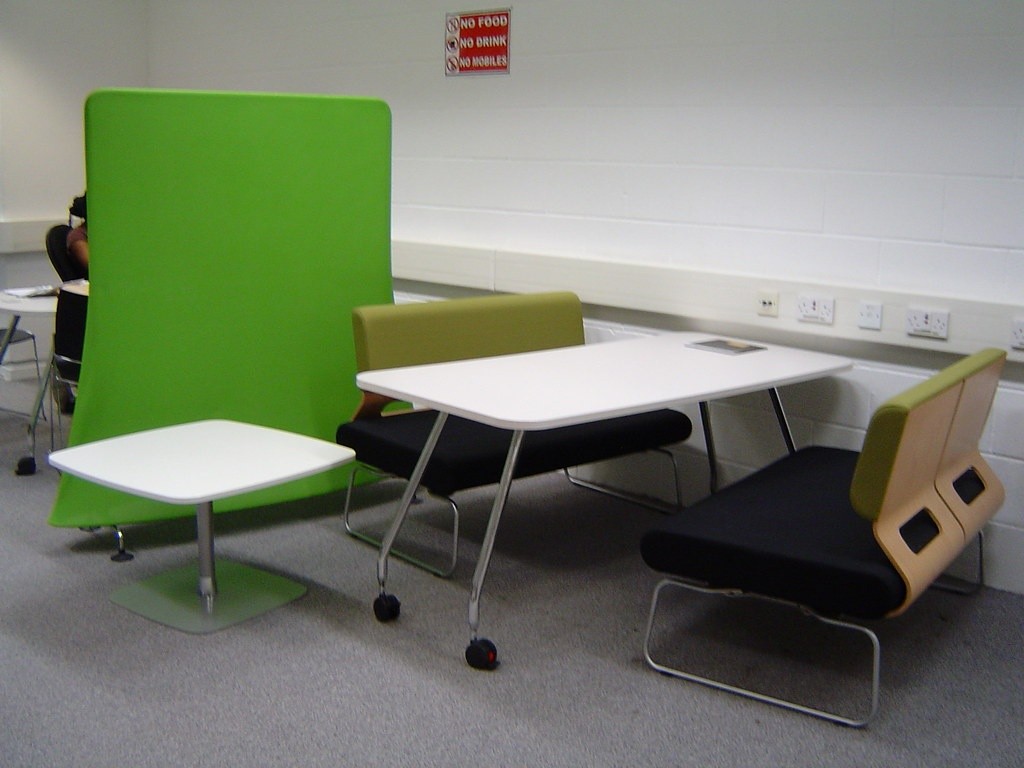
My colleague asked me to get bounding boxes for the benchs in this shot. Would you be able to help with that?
[334,292,693,577]
[643,345,1006,727]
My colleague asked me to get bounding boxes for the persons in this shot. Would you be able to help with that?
[60,190,88,279]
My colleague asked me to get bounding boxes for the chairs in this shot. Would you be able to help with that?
[0,328,47,422]
[43,224,89,389]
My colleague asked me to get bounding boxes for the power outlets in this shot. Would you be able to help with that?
[858,302,881,329]
[756,293,778,318]
[1010,322,1024,350]
[797,296,833,325]
[906,307,949,339]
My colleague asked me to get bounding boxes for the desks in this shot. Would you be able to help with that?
[356,335,853,669]
[0,286,58,475]
[49,418,356,635]
